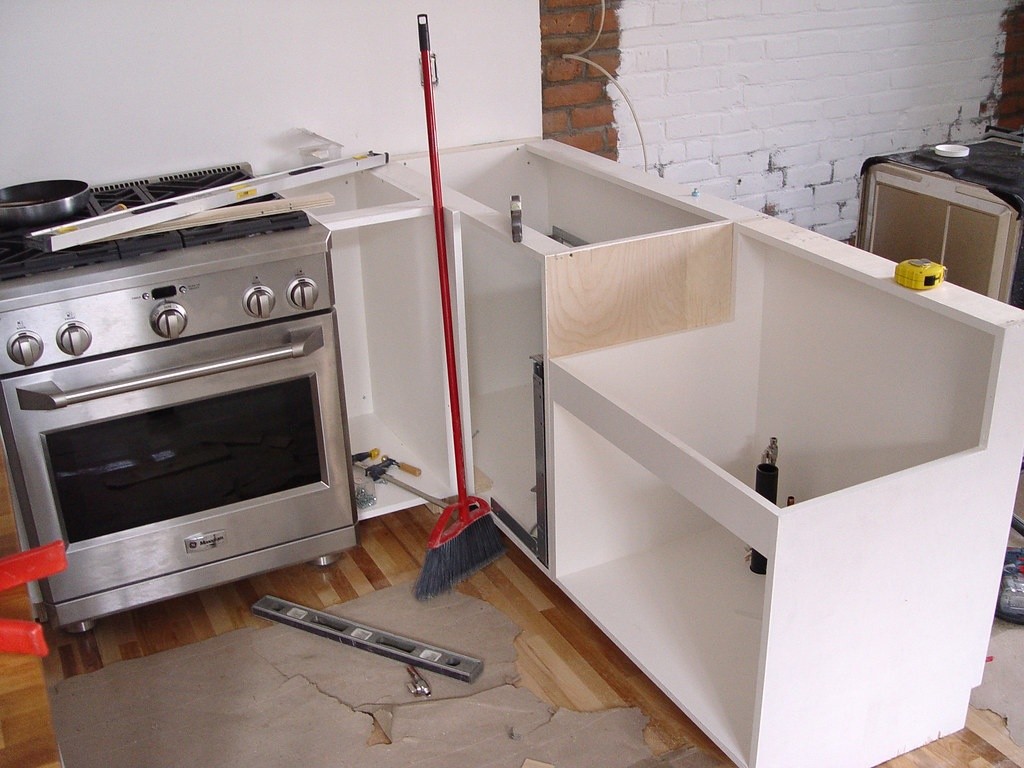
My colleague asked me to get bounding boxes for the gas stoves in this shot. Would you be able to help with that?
[1,158,336,383]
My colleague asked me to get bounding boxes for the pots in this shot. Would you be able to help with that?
[0,180,91,226]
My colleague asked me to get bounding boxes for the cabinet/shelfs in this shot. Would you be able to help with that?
[281,138,1024,768]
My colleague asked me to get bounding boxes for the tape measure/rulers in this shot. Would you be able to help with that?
[894,257,949,291]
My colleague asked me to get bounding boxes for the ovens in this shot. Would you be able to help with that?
[2,309,361,637]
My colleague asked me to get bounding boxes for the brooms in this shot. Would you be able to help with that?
[412,14,512,603]
